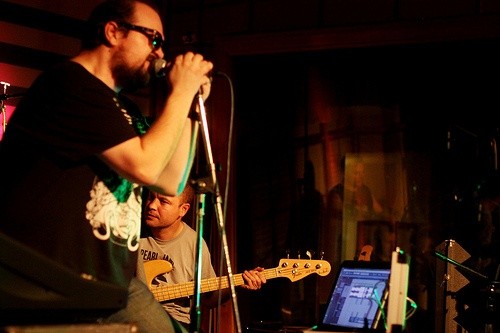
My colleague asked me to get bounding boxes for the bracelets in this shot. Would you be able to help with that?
[187,109,202,120]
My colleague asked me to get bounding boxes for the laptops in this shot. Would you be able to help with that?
[316,259,392,333]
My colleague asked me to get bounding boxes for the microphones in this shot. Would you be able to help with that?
[151,59,218,78]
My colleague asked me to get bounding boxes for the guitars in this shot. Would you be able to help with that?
[138,251,332,302]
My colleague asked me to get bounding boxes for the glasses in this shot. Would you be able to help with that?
[118,20,165,50]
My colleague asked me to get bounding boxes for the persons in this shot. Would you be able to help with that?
[135,180,265,333]
[0,0,212,333]
